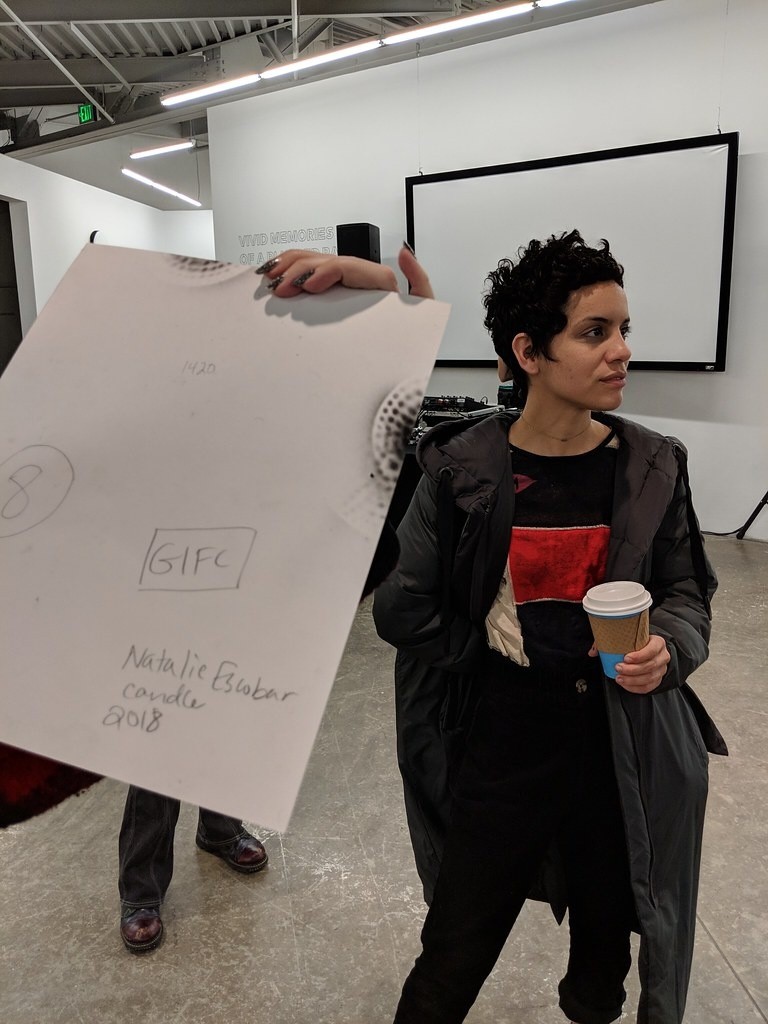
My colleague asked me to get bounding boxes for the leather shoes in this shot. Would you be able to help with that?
[119,901,164,951]
[195,829,269,874]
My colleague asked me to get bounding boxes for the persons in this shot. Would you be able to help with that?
[370,229,732,1023]
[0,240,436,956]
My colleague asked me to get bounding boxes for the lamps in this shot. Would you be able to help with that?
[160,0,539,106]
[120,167,202,207]
[129,138,196,159]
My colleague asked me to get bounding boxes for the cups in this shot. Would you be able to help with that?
[582,581,653,679]
[498,385,513,410]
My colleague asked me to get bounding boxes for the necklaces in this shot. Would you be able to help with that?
[521,412,592,442]
